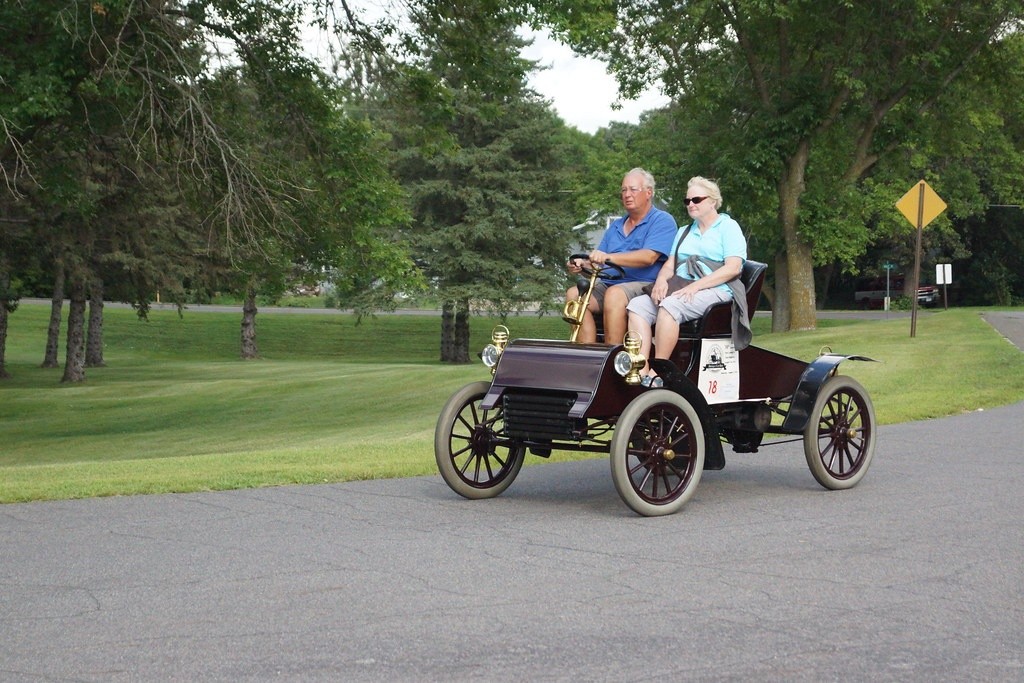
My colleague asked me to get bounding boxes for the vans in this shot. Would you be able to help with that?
[854,273,940,310]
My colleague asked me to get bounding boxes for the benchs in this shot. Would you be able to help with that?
[592,260,768,338]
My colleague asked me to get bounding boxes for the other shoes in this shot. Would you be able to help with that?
[641,374,663,388]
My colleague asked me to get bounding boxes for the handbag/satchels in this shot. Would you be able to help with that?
[641,274,697,308]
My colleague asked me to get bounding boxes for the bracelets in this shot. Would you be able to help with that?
[606,253,610,261]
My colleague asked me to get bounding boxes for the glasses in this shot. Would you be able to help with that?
[683,196,711,205]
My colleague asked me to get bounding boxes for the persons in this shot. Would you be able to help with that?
[566,167,678,344]
[626,176,747,387]
